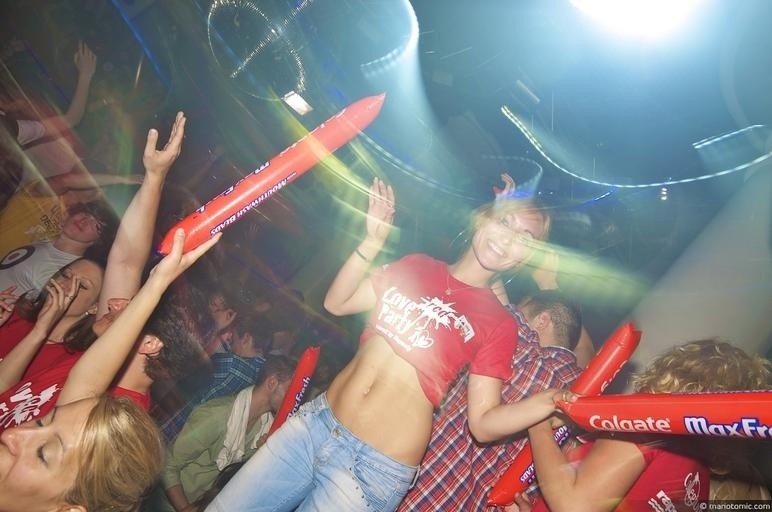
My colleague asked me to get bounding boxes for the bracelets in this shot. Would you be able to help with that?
[355,248,375,264]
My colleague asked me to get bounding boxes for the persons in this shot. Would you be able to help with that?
[500,336,772,512]
[0,35,352,511]
[203,174,587,512]
[396,175,598,512]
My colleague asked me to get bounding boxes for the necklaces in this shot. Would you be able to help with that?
[443,265,488,298]
